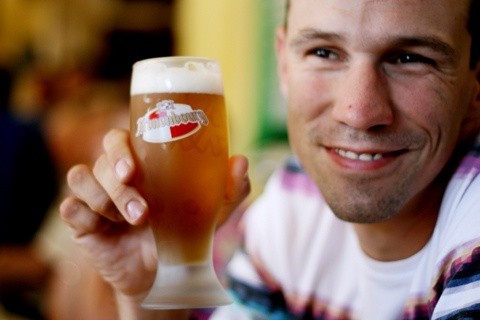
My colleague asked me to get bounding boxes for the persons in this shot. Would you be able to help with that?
[58,0,480,320]
[0,96,117,319]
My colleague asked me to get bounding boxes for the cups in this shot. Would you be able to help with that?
[129,56,233,309]
